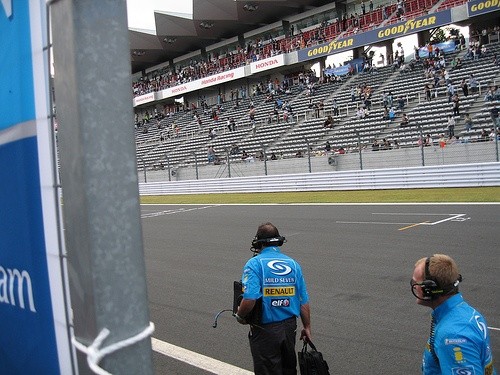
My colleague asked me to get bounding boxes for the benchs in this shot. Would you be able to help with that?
[133,0,500,172]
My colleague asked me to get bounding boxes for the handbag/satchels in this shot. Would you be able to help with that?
[298,338,330,375]
[232,281,262,324]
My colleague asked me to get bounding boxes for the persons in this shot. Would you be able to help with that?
[235,223,312,375]
[410,254,497,375]
[54,0,500,170]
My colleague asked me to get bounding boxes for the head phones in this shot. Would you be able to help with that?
[252,235,285,248]
[420,256,462,300]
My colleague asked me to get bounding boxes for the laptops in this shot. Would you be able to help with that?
[233,280,264,329]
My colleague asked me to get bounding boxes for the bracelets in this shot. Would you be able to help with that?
[236,313,245,322]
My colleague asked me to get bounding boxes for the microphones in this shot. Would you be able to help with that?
[411,280,435,300]
[250,247,258,253]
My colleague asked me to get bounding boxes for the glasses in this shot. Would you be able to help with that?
[410,279,422,286]
